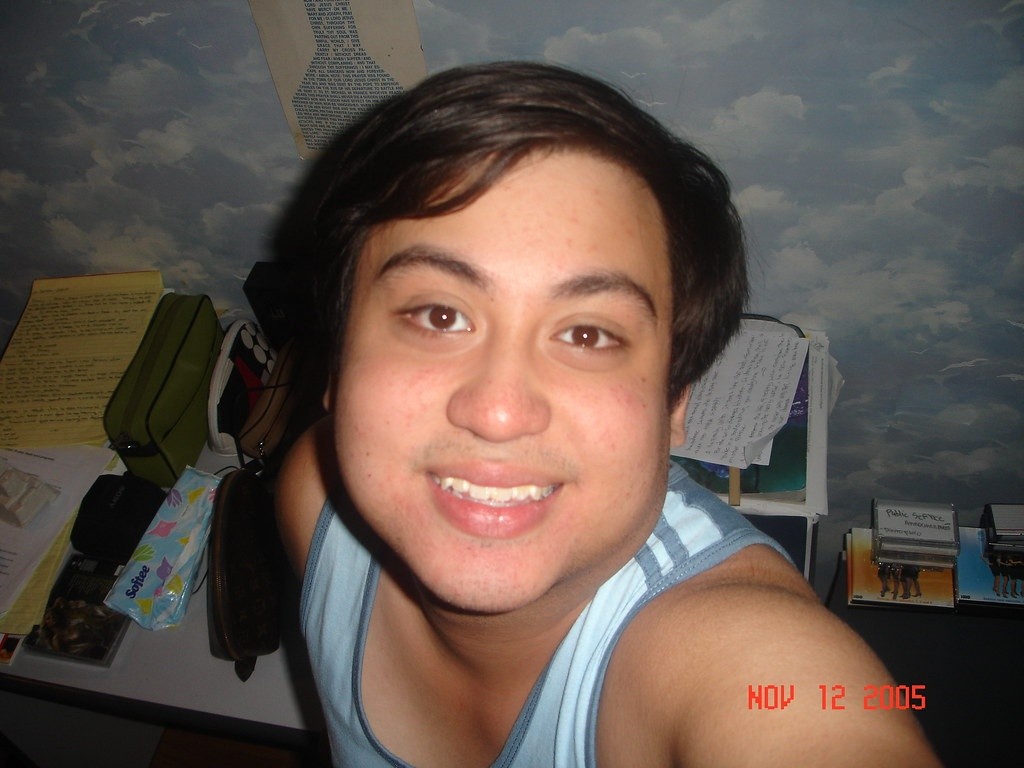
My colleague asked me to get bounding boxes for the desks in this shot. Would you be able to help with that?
[1,315,820,764]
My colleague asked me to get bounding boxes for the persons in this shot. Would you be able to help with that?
[877,563,922,601]
[982,552,1024,599]
[276,60,942,768]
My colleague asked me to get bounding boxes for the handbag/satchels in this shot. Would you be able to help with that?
[102,293,228,488]
[239,337,321,461]
[208,318,279,457]
[101,466,225,630]
[207,466,302,683]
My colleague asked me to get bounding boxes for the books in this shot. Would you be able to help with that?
[0,271,176,443]
[668,332,845,518]
[0,445,132,669]
